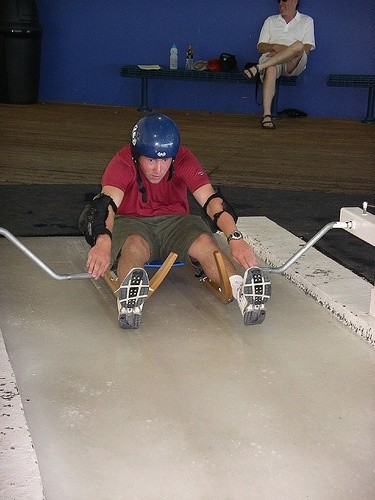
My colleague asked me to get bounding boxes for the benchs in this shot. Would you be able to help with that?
[120,65,297,121]
[326,73,375,125]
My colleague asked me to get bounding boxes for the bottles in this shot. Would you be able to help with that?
[170,45,178,69]
[186,45,194,71]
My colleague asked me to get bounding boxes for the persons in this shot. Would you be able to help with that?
[243,0,315,129]
[86,113,271,330]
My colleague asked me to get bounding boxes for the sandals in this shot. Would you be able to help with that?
[243,65,259,79]
[261,114,275,128]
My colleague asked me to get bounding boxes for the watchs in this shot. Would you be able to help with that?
[226,230,244,244]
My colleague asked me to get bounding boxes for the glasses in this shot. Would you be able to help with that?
[277,0,288,3]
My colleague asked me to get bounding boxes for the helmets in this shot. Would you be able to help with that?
[131,113,180,158]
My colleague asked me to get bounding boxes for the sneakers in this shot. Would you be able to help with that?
[228,266,272,326]
[116,268,149,328]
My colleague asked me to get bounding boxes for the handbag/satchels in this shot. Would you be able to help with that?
[245,62,260,78]
[218,53,238,73]
